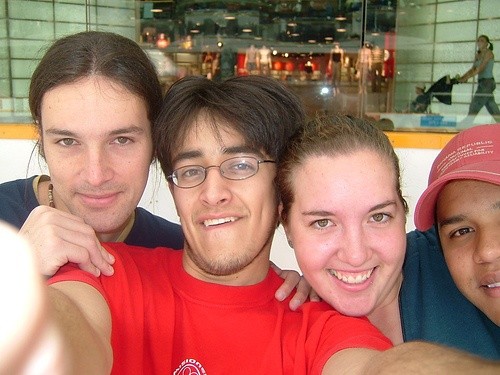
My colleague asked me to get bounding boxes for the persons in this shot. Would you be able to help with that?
[458,35,500,130]
[325,41,350,96]
[356,41,382,96]
[0,77,500,375]
[414,124,500,327]
[402,83,426,113]
[245,45,272,77]
[276,113,500,361]
[0,30,319,311]
[204,51,220,74]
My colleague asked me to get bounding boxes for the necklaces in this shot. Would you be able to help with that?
[49,184,56,208]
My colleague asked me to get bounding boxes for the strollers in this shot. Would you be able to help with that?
[394,74,467,113]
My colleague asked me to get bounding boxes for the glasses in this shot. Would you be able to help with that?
[166,156,276,188]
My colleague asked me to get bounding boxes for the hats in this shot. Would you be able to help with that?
[414,123,500,231]
